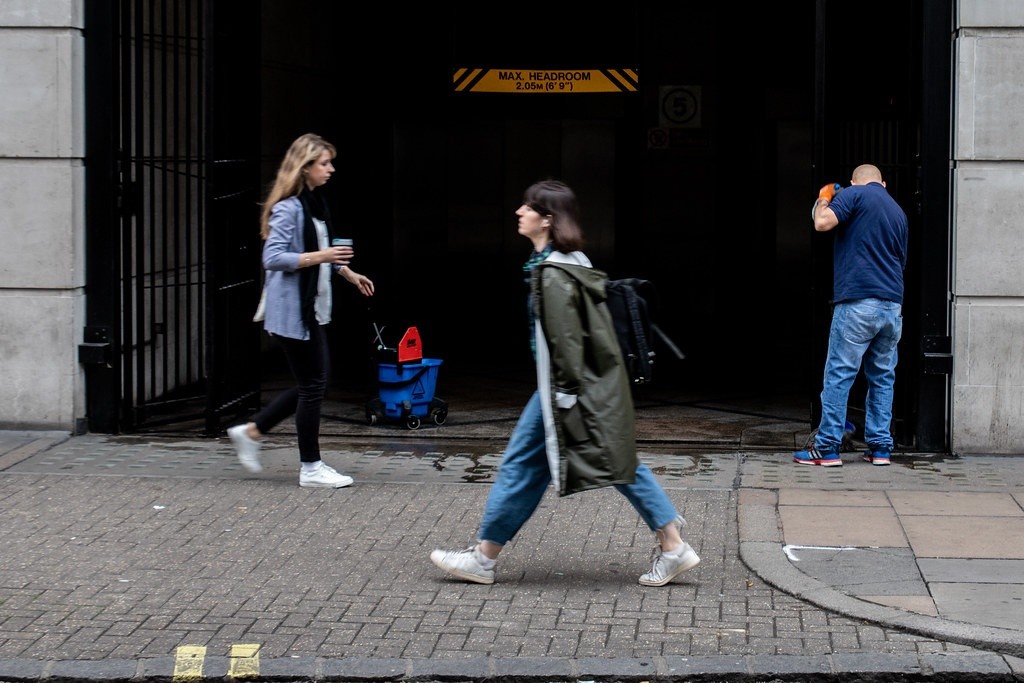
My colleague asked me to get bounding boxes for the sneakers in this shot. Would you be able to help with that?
[227,425,263,475]
[793,445,842,467]
[638,545,700,587]
[430,547,497,585]
[864,446,890,466]
[299,462,353,489]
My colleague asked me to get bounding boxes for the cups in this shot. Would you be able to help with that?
[331,238,353,269]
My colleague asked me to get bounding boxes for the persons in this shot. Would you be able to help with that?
[430,180,701,586]
[793,164,909,466]
[228,135,374,488]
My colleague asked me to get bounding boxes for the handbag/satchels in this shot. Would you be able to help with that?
[606,277,656,393]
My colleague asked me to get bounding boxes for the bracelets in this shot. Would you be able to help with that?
[305,253,311,267]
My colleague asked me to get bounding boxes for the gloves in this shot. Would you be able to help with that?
[812,183,843,219]
